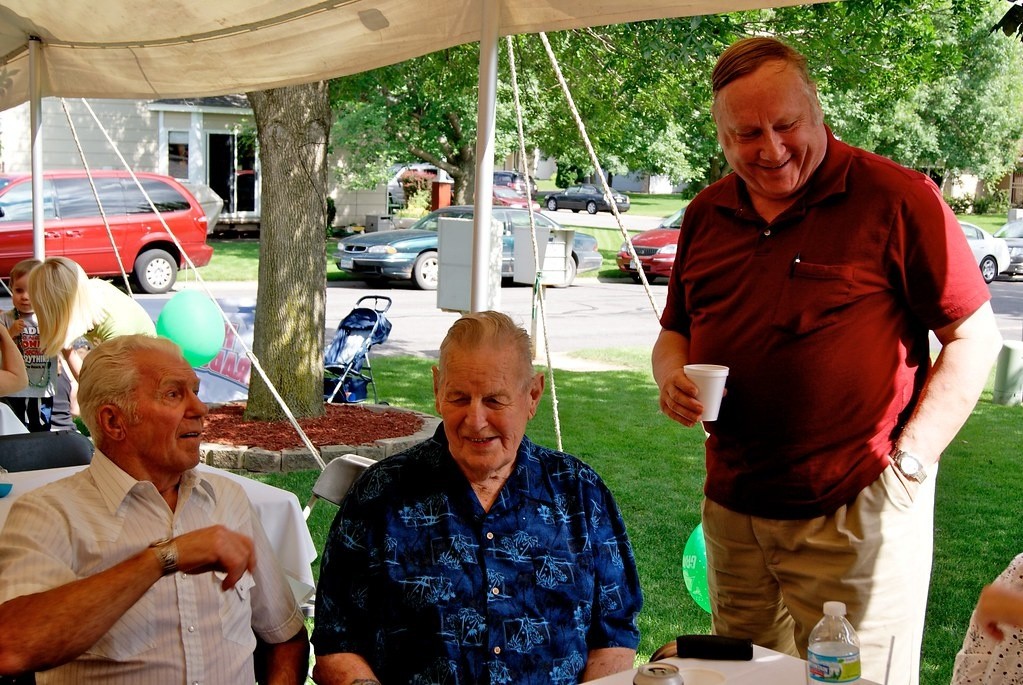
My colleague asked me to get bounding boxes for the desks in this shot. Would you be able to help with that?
[0,465,91,529]
[571,644,882,685]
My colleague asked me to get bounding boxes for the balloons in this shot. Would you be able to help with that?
[155,289,225,367]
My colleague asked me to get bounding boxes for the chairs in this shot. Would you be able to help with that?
[301,452,380,678]
[0,430,94,473]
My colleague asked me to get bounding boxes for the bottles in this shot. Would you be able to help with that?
[807,600,862,685]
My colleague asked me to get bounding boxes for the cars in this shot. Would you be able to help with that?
[543,184,630,216]
[333,206,603,290]
[615,206,687,283]
[493,186,540,213]
[224,170,254,211]
[993,219,1023,276]
[958,221,1011,284]
[493,171,537,198]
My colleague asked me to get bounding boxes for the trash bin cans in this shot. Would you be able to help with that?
[436,217,503,312]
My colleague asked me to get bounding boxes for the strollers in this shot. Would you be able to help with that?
[323,296,393,404]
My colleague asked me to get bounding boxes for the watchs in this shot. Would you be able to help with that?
[889,446,928,485]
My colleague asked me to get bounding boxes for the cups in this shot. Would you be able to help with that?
[684,364,729,421]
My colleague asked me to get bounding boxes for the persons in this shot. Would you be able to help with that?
[322,311,643,685]
[974,580,1023,643]
[0,256,159,433]
[650,38,1001,657]
[0,334,315,685]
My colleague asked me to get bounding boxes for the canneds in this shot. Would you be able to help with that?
[633,662,684,685]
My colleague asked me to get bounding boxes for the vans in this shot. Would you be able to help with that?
[0,169,213,294]
[385,163,455,215]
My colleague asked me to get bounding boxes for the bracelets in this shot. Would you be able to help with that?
[349,679,379,685]
[147,536,178,575]
